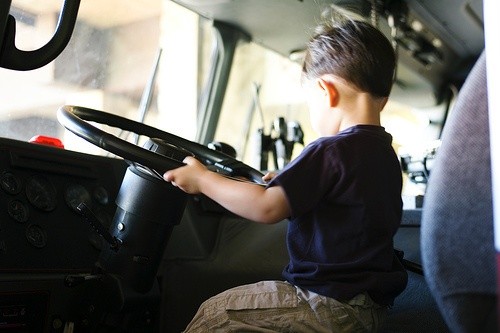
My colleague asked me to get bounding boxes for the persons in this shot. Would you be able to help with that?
[162,22,408,333]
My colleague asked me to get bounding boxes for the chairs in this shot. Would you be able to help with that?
[368,51,500,332]
